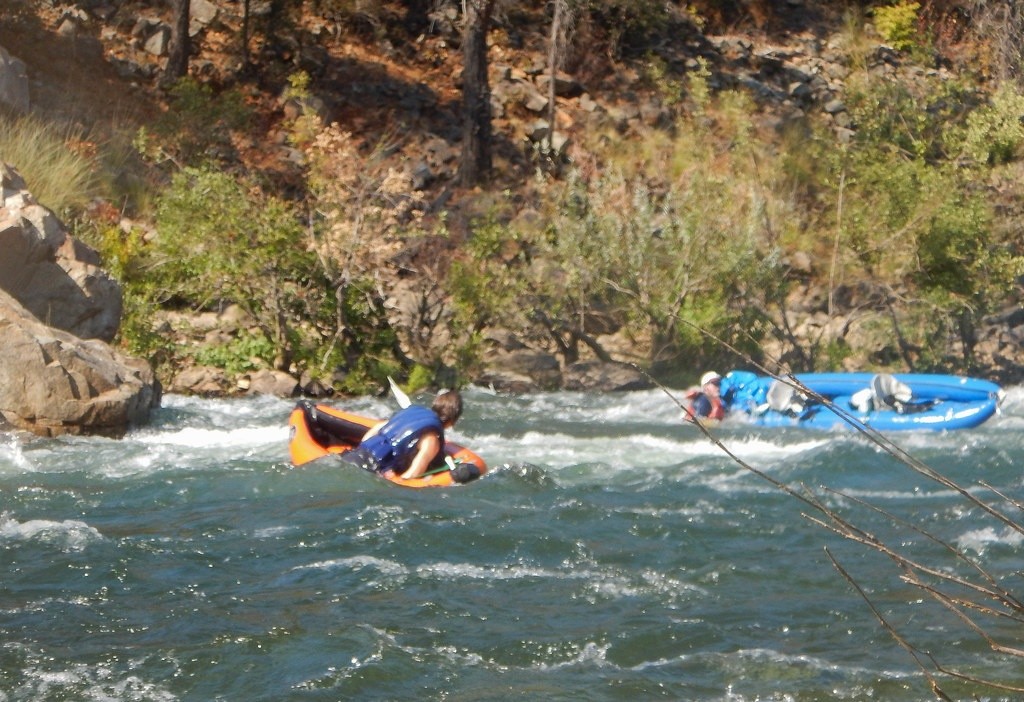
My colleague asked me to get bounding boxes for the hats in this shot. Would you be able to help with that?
[700,371,720,387]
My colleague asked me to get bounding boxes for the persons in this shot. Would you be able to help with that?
[685,370,727,423]
[344,390,463,479]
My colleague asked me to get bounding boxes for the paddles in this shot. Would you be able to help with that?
[386,374,456,471]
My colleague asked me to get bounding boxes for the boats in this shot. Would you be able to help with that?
[286,394,488,490]
[683,369,1002,431]
